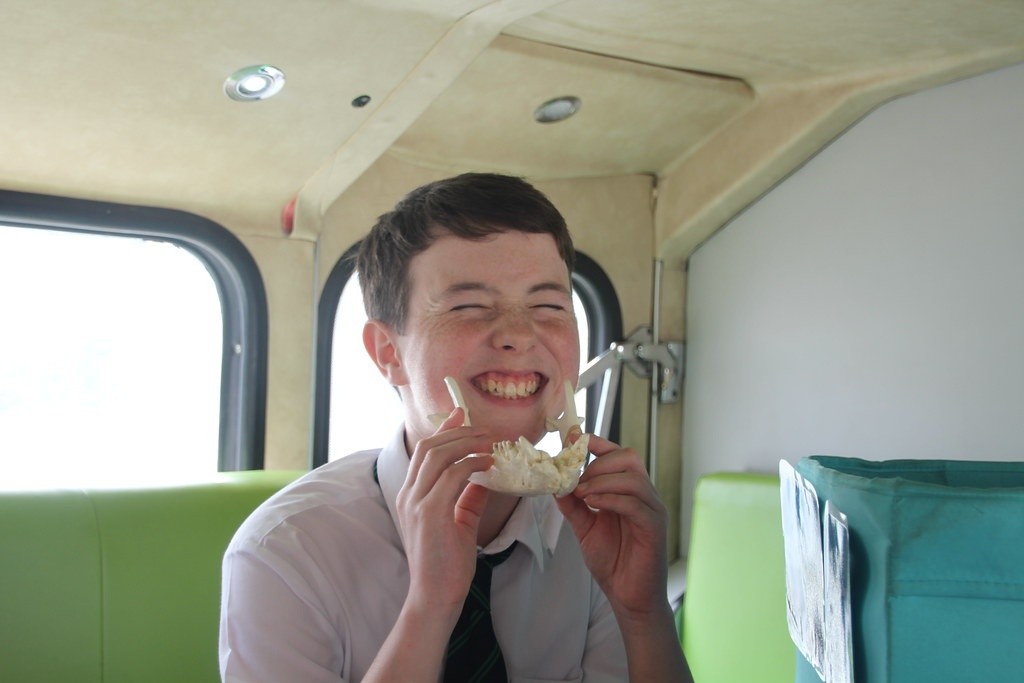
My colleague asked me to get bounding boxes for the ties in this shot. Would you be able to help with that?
[442,542,520,683]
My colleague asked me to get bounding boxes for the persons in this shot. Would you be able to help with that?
[218,172,695,683]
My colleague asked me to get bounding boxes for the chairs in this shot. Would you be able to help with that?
[664,474,801,682]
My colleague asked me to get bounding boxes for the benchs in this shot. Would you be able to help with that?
[0,466,306,683]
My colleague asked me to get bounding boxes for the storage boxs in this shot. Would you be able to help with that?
[778,457,1024,683]
